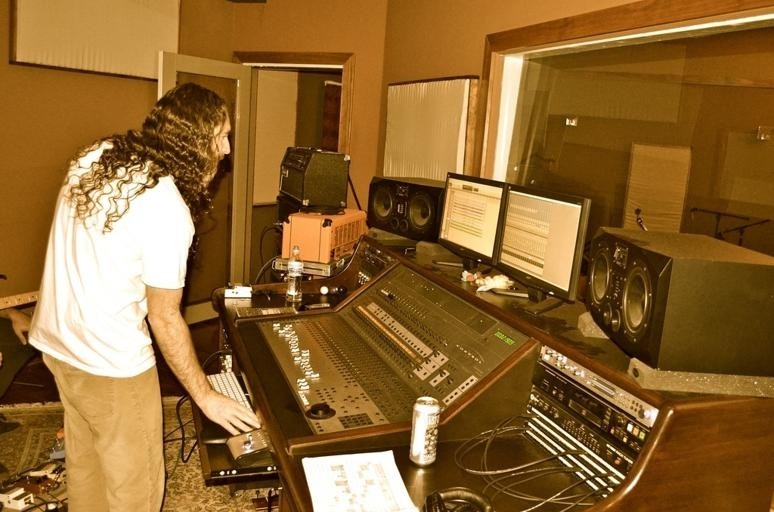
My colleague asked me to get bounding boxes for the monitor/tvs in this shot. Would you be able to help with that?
[432,172,509,276]
[493,183,592,314]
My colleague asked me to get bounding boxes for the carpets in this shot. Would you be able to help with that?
[1,389,280,512]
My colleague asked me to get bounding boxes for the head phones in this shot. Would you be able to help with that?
[422,486,492,512]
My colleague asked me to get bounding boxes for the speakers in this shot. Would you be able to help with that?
[584,226,774,378]
[366,175,446,243]
[279,146,350,207]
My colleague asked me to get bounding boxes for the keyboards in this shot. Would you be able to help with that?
[206,372,254,413]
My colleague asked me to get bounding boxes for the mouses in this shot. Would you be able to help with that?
[200,429,227,444]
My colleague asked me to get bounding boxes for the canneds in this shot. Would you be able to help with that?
[408,396,441,467]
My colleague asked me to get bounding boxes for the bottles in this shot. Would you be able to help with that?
[286,246,304,303]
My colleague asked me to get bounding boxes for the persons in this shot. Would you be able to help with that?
[0,270,36,438]
[27,81,262,511]
